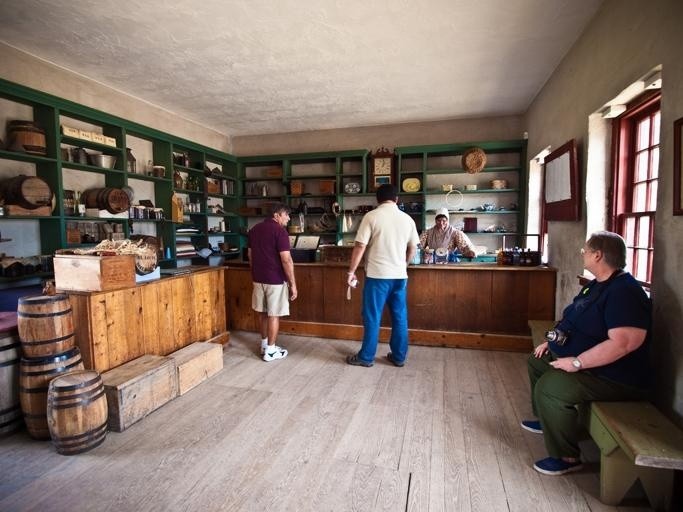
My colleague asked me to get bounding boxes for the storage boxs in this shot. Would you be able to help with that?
[165,340,224,395]
[100,354,177,432]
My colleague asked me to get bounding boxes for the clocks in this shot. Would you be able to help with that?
[366,145,396,192]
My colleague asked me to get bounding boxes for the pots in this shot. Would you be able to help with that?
[220,180,235,195]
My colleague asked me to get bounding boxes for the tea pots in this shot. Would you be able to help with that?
[247,183,258,196]
[181,150,192,167]
[480,203,495,211]
[68,144,90,166]
[259,184,269,196]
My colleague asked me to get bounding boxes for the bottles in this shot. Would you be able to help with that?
[496,247,534,266]
[398,199,404,212]
[184,197,200,212]
[146,160,153,177]
[172,168,201,191]
[126,148,136,173]
[73,191,85,217]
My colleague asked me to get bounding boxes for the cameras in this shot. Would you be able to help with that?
[545,327,569,347]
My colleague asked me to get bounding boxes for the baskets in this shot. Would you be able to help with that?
[462,147,487,174]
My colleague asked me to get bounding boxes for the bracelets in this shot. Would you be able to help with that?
[347,272,354,275]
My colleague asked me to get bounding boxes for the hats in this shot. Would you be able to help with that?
[435,208,448,219]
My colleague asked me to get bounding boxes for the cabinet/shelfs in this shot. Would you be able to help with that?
[239,148,367,259]
[0,78,240,285]
[394,140,528,263]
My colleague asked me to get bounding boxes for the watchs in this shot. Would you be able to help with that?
[572,357,581,371]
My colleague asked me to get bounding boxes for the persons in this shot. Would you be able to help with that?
[346,184,420,367]
[417,210,476,257]
[521,232,652,476]
[248,204,298,362]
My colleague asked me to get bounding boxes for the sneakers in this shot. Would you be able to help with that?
[346,355,374,367]
[261,345,281,354]
[387,352,404,366]
[263,350,288,361]
[521,419,544,435]
[533,457,583,476]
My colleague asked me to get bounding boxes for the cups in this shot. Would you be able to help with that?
[41,255,52,272]
[346,205,372,212]
[89,154,117,168]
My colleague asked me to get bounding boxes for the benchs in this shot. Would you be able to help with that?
[528,320,683,511]
